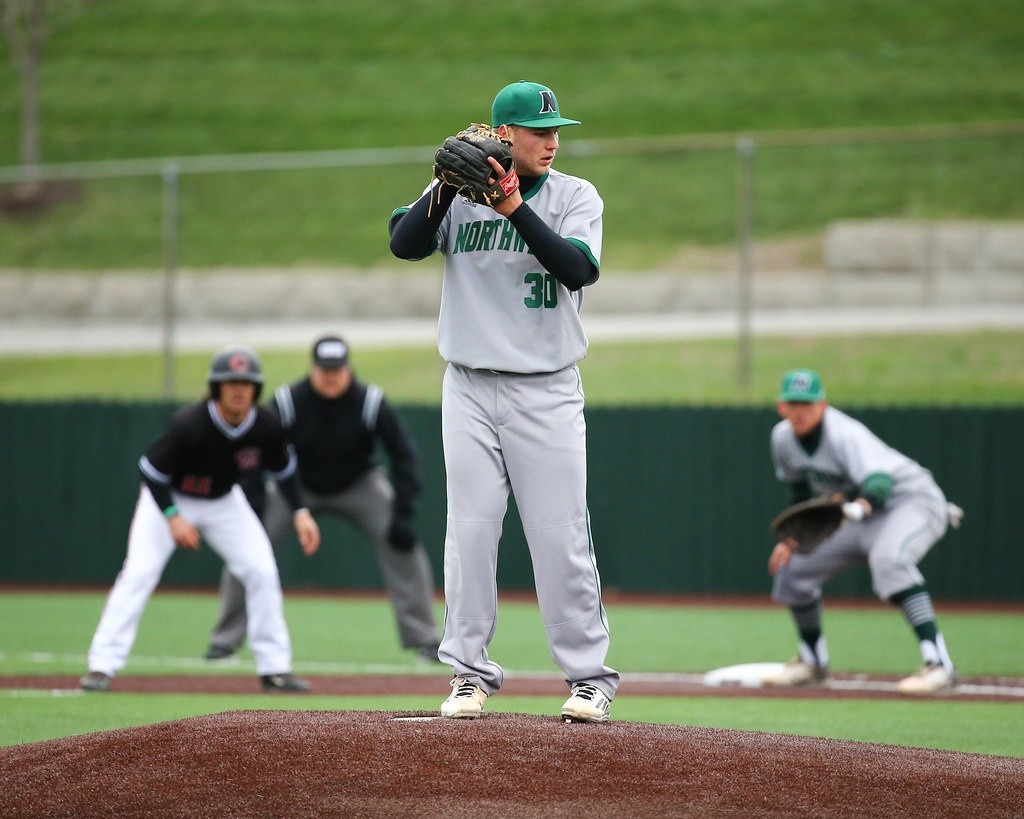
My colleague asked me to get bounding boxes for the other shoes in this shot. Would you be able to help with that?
[763,655,829,689]
[418,644,439,662]
[207,646,228,658]
[897,664,957,696]
[79,671,111,690]
[262,674,309,691]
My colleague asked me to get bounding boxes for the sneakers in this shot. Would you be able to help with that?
[561,683,611,722]
[441,677,487,719]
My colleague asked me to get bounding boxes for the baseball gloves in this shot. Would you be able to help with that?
[434,122,521,208]
[769,492,850,555]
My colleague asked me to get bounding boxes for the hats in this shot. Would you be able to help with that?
[780,369,821,402]
[491,80,581,128]
[313,338,348,368]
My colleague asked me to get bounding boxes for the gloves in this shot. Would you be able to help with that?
[385,507,418,551]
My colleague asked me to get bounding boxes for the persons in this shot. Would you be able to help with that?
[78,344,312,694]
[388,78,622,724]
[761,368,957,696]
[208,338,445,665]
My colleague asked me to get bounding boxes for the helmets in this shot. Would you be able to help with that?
[207,346,263,402]
